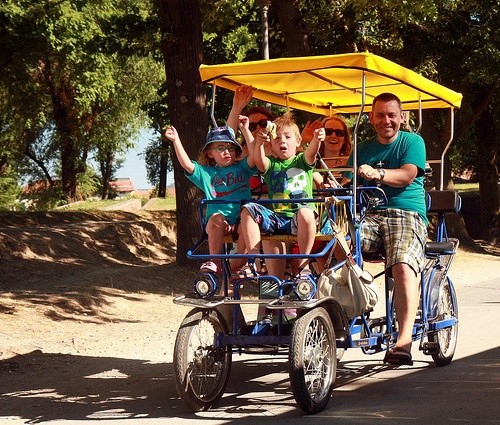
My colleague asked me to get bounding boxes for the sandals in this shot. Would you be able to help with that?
[230,266,266,279]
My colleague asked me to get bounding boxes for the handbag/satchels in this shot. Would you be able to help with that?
[316,236,379,319]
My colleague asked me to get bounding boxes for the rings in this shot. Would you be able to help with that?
[367,173,371,176]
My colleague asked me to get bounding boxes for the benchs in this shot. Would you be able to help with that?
[426,191,462,257]
[222,201,347,242]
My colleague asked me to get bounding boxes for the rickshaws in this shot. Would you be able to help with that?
[171,51,480,416]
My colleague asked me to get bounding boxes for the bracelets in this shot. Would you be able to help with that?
[375,167,385,184]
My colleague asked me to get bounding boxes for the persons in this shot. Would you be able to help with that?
[324,93,429,363]
[224,85,326,333]
[165,114,256,297]
[296,115,353,283]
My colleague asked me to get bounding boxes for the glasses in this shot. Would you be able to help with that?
[249,119,268,132]
[324,127,347,137]
[209,145,237,153]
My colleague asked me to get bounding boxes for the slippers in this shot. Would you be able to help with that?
[386,347,412,361]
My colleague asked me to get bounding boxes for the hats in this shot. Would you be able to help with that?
[202,126,243,157]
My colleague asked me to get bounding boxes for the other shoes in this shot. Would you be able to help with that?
[199,260,223,279]
[262,310,275,321]
